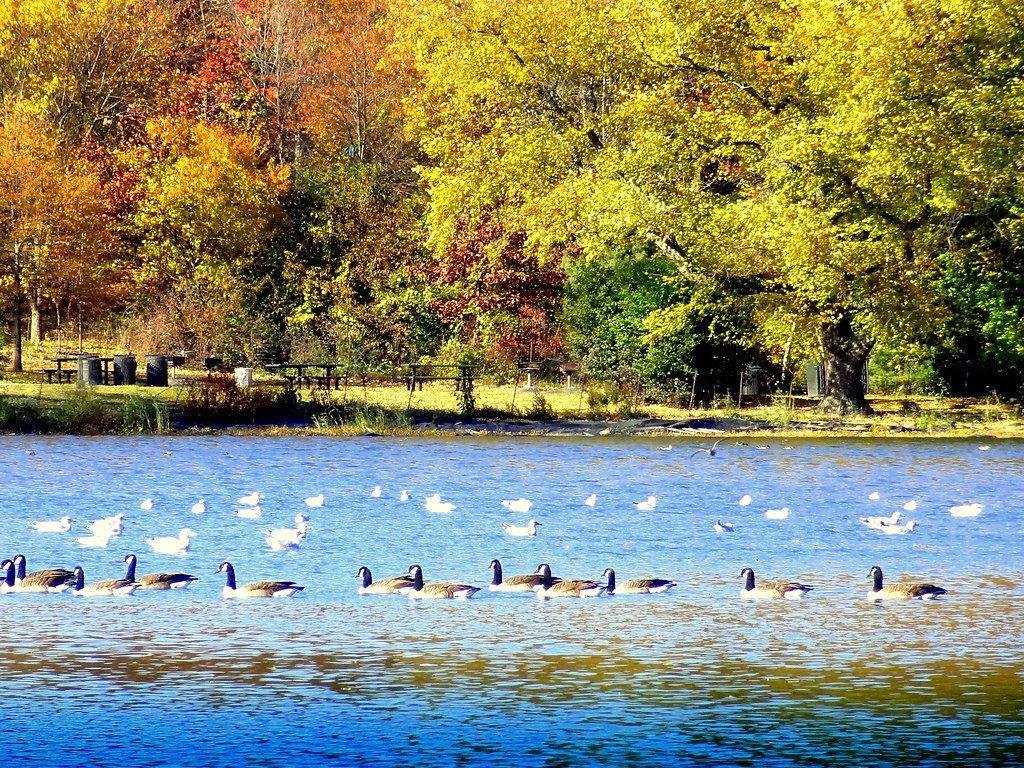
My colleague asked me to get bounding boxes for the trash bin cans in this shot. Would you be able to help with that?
[145,353,169,387]
[82,357,102,384]
[112,355,136,384]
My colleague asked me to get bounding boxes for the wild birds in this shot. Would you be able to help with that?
[0,439,1024,606]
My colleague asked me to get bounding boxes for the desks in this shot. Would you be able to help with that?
[408,364,481,392]
[266,364,345,390]
[51,358,114,386]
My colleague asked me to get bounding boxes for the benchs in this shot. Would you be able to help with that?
[298,375,349,390]
[57,369,114,384]
[42,369,64,384]
[402,376,460,392]
[409,375,483,391]
[284,376,327,390]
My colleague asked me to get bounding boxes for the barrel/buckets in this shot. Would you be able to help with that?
[147,356,167,386]
[114,355,135,384]
[80,357,101,384]
[235,368,252,387]
